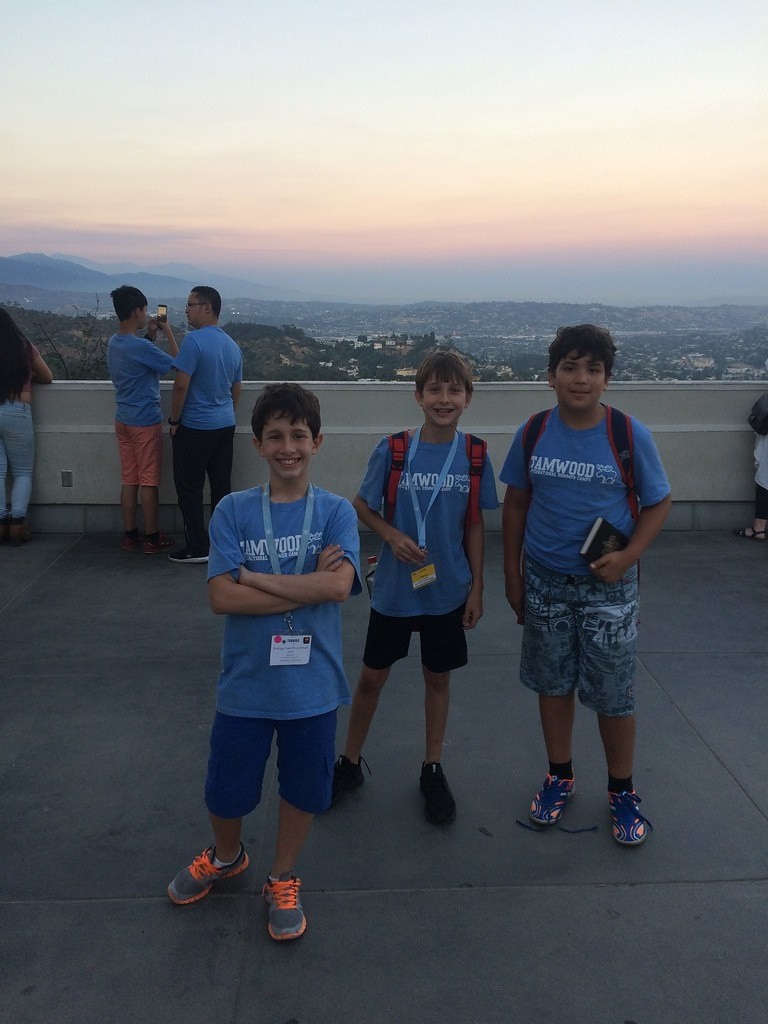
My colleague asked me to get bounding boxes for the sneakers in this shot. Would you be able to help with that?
[516,773,598,833]
[609,788,653,845]
[328,754,371,811]
[420,761,457,826]
[168,546,209,562]
[262,871,306,941]
[143,537,176,553]
[122,533,144,551]
[168,841,249,904]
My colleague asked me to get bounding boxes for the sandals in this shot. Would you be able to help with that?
[733,526,766,540]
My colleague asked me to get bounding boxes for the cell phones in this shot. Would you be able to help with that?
[157,305,167,328]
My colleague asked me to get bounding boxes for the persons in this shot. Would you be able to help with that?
[103,284,180,555]
[732,356,768,543]
[0,306,53,547]
[167,285,245,565]
[329,348,501,828]
[498,323,673,848]
[165,381,365,942]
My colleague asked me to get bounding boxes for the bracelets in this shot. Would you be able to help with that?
[168,416,182,425]
[144,333,157,342]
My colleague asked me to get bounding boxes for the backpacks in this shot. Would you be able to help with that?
[748,393,768,435]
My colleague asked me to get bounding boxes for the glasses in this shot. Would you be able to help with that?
[186,302,209,308]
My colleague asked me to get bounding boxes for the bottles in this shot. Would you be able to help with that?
[367,556,378,596]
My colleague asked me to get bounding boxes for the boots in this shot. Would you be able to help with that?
[0,519,10,546]
[10,522,32,546]
